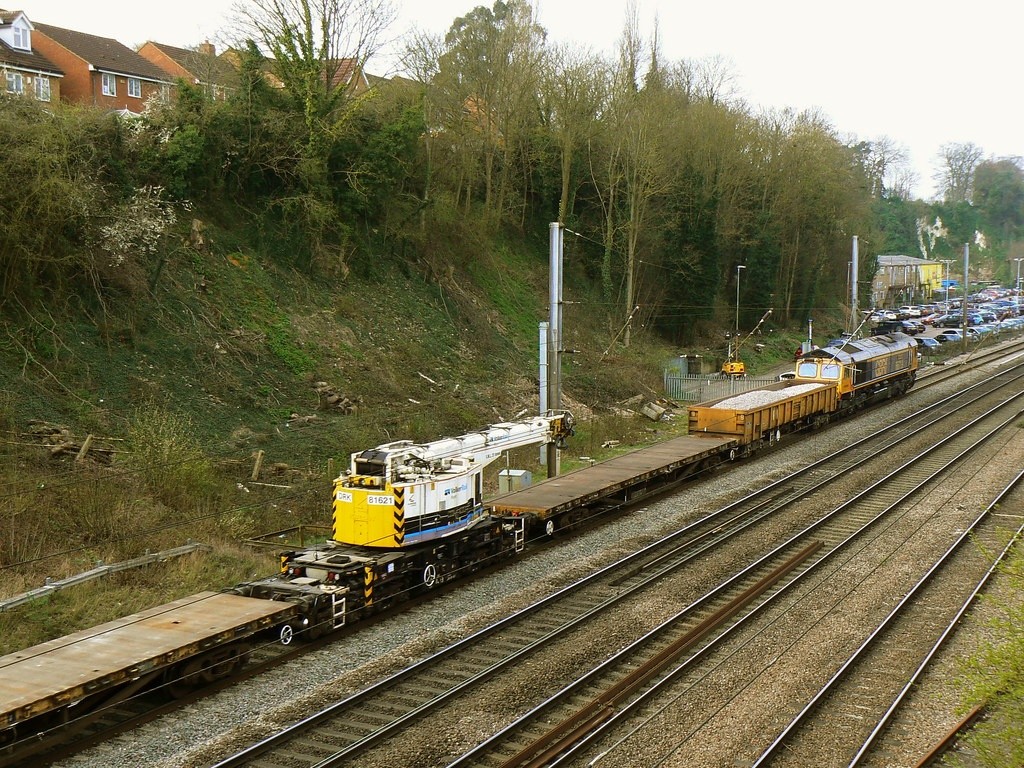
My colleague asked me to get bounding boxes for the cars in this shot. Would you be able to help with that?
[867,287,1024,351]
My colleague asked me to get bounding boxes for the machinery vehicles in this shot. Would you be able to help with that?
[721,358,744,380]
[330,408,578,546]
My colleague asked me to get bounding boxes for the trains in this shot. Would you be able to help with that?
[1,332,919,731]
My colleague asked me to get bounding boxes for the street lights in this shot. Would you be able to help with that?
[1013,257,1024,290]
[939,259,958,301]
[735,265,746,360]
